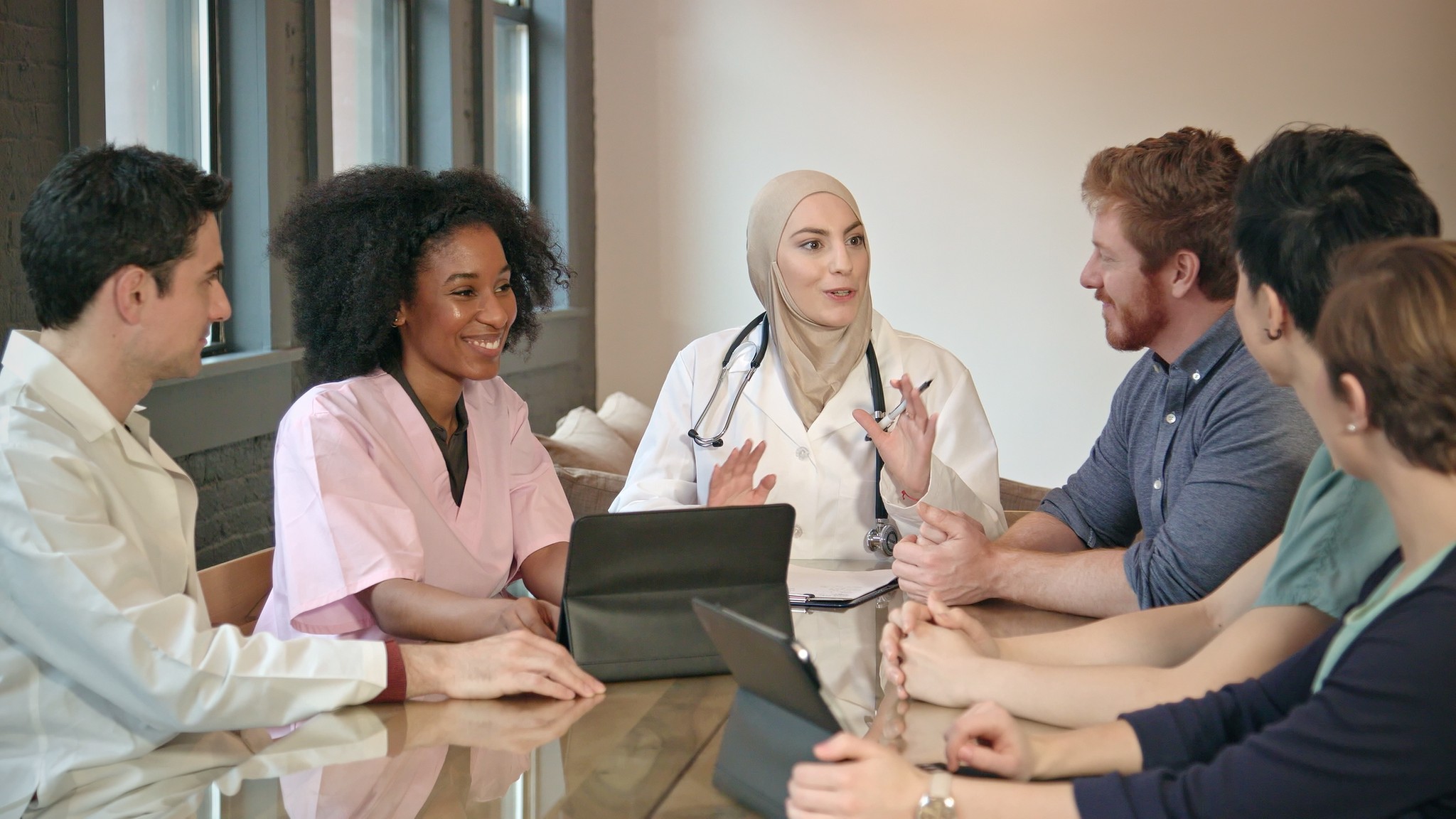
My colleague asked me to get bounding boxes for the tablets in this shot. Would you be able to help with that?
[554,509,801,679]
[695,598,867,752]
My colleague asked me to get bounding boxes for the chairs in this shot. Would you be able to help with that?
[197,547,275,637]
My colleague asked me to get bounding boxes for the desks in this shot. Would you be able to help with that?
[163,561,1105,818]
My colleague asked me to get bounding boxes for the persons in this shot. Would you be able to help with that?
[250,164,580,649]
[3,143,621,817]
[877,123,1448,727]
[881,128,1336,616]
[610,174,1003,562]
[2,684,610,818]
[262,712,533,819]
[779,236,1456,819]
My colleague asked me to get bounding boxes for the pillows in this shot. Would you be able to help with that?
[552,405,634,473]
[554,463,628,519]
[531,432,621,475]
[598,391,653,452]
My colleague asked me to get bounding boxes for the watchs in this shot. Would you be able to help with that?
[913,768,954,819]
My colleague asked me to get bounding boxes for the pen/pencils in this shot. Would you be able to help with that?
[864,378,933,442]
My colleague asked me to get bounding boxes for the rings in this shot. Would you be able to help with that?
[905,408,915,418]
